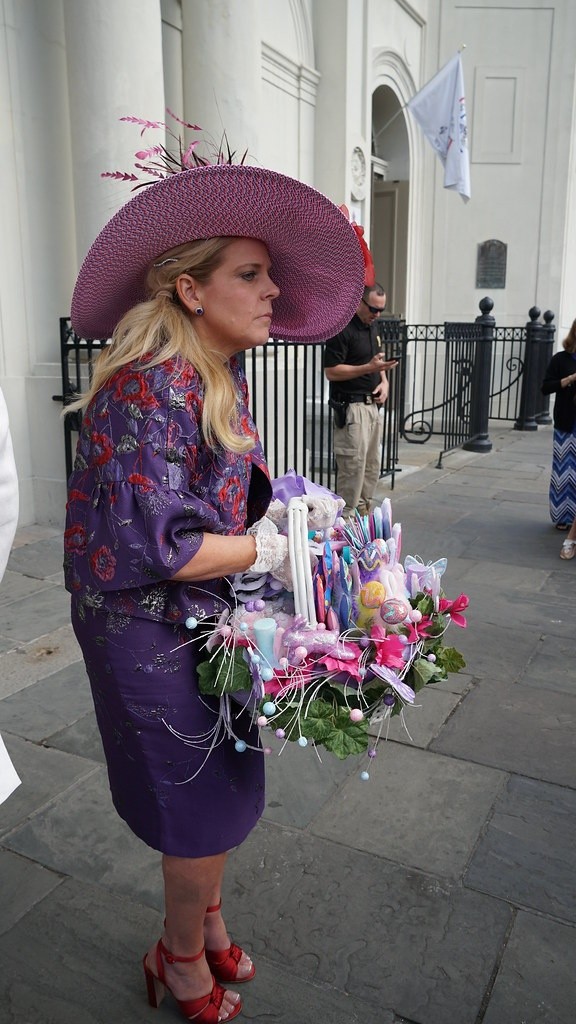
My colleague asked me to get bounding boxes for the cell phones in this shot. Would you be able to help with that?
[387,356,402,361]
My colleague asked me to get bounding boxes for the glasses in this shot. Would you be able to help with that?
[360,298,387,314]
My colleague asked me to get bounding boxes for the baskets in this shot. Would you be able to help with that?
[227,496,426,723]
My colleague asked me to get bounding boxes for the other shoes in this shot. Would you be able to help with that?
[559,539,576,560]
[556,522,569,531]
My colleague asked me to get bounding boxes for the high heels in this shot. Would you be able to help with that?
[163,896,257,983]
[142,937,244,1024]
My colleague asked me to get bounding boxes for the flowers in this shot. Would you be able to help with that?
[161,577,468,785]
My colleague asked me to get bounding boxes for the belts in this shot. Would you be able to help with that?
[333,392,374,405]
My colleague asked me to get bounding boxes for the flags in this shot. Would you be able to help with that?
[407,51,473,205]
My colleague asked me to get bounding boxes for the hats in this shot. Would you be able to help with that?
[70,164,366,344]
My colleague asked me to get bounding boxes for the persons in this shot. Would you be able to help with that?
[61,166,367,1024]
[323,280,398,519]
[540,321,576,561]
